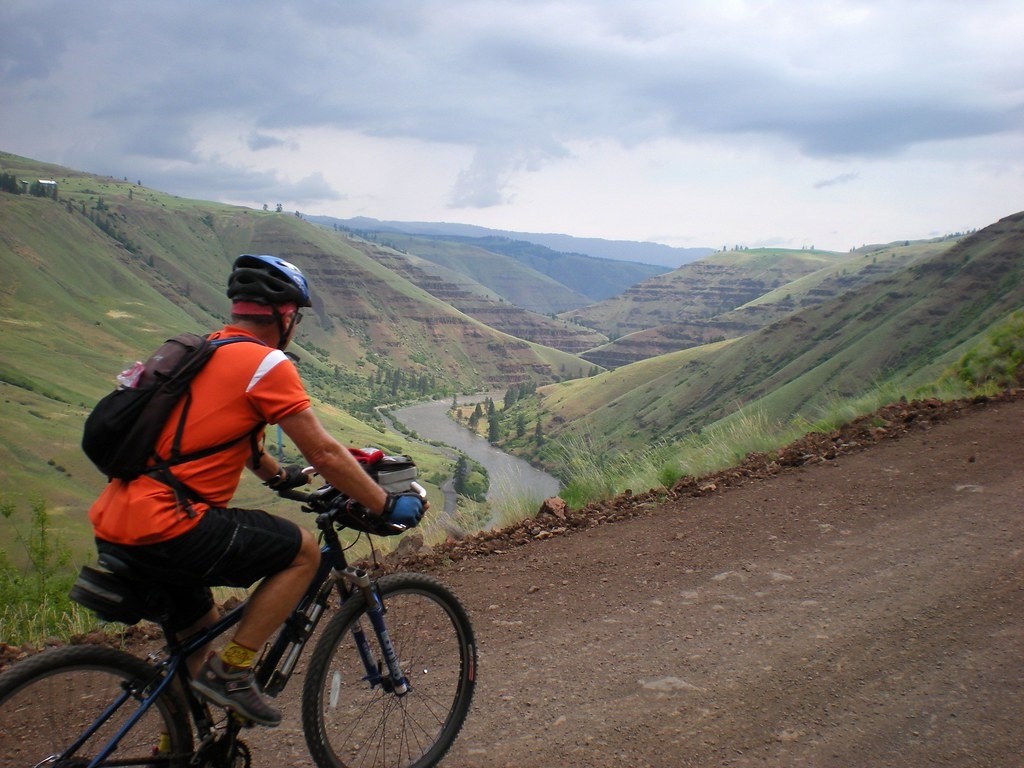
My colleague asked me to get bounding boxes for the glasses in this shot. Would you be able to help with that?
[293,312,304,325]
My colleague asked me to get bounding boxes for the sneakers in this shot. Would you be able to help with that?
[189,652,281,727]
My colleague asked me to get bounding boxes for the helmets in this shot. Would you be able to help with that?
[227,253,312,307]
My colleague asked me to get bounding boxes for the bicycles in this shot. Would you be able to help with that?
[0,452,481,768]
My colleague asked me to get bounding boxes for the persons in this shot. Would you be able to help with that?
[87,254,430,768]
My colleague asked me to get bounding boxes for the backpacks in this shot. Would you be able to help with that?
[82,333,268,480]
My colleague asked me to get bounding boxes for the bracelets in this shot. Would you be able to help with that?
[261,466,284,486]
